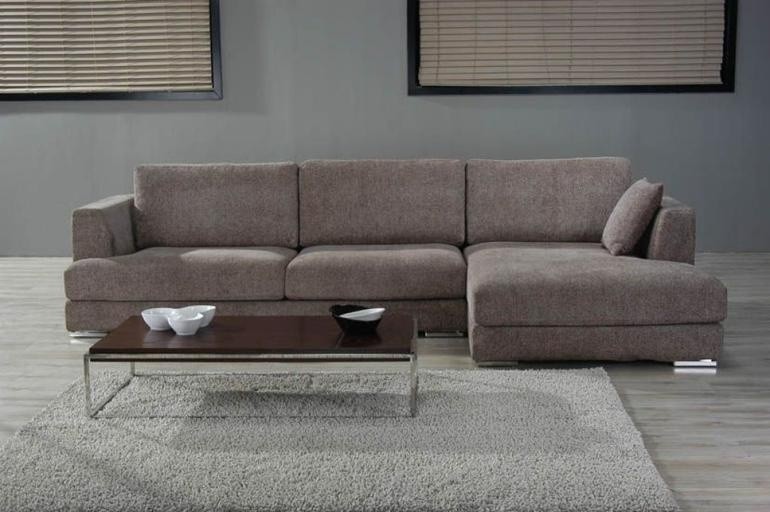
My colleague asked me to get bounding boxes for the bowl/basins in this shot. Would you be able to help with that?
[330,302,386,333]
[332,329,382,348]
[141,305,216,335]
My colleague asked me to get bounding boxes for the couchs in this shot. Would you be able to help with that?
[58,155,729,367]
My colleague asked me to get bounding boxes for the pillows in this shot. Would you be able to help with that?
[600,175,666,258]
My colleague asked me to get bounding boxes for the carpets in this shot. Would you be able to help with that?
[2,349,682,507]
